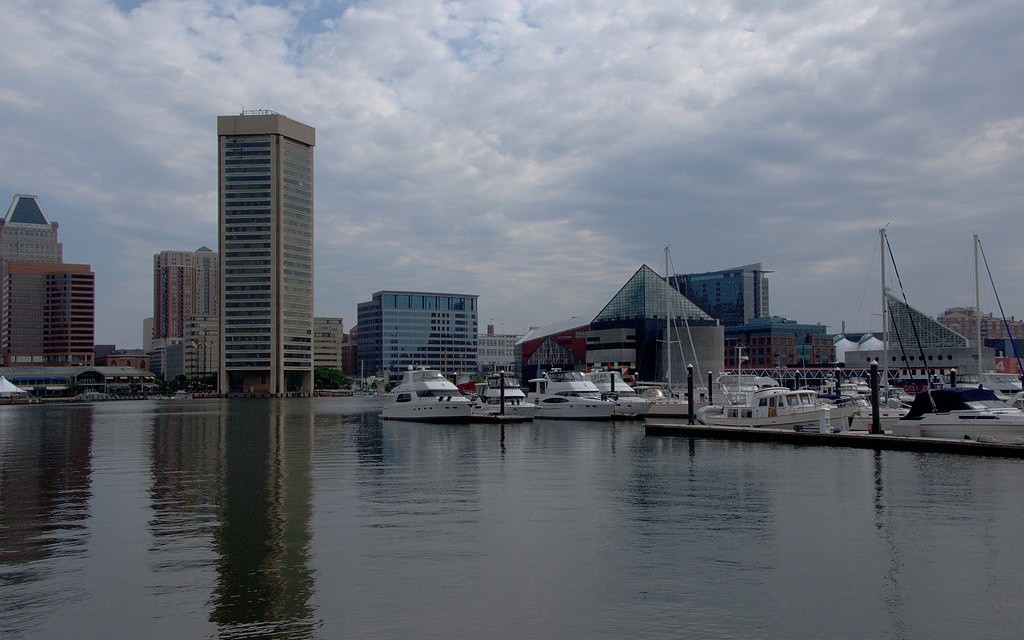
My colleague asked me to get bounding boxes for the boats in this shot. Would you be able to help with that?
[469,371,543,423]
[526,370,616,421]
[697,377,915,432]
[895,386,1024,445]
[378,366,475,421]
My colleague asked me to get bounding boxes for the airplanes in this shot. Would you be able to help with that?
[587,371,654,418]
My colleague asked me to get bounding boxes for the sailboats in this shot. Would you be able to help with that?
[354,359,377,396]
[828,225,932,431]
[633,244,707,415]
